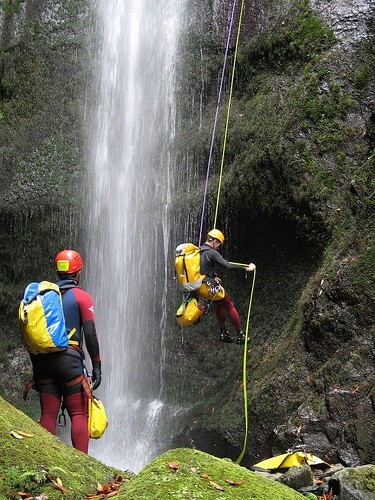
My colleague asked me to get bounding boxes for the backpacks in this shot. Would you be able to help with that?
[175,243,205,291]
[18,281,83,354]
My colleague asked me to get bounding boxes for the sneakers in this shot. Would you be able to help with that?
[236,333,251,345]
[219,330,235,343]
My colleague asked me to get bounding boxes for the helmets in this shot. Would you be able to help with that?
[208,228,224,244]
[54,249,83,274]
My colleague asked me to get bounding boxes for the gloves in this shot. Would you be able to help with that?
[90,362,101,391]
[247,263,256,271]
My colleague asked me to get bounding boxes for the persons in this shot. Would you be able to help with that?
[175,229,251,346]
[18,250,102,455]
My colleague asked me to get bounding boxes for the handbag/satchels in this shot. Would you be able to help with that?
[87,387,108,439]
[174,296,206,327]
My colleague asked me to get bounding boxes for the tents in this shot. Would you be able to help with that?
[250,451,332,472]
